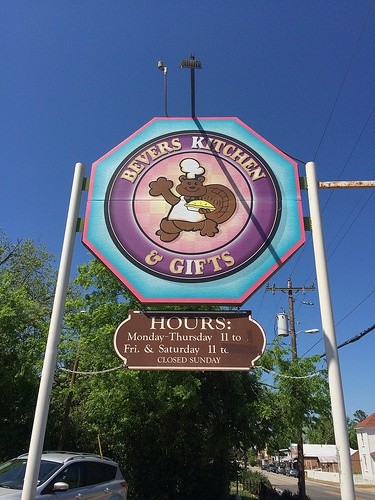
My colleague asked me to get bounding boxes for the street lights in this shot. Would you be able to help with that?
[292,328,322,497]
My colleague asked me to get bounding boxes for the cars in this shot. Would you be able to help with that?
[261,463,298,478]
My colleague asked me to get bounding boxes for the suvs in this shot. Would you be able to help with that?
[0,451,128,500]
